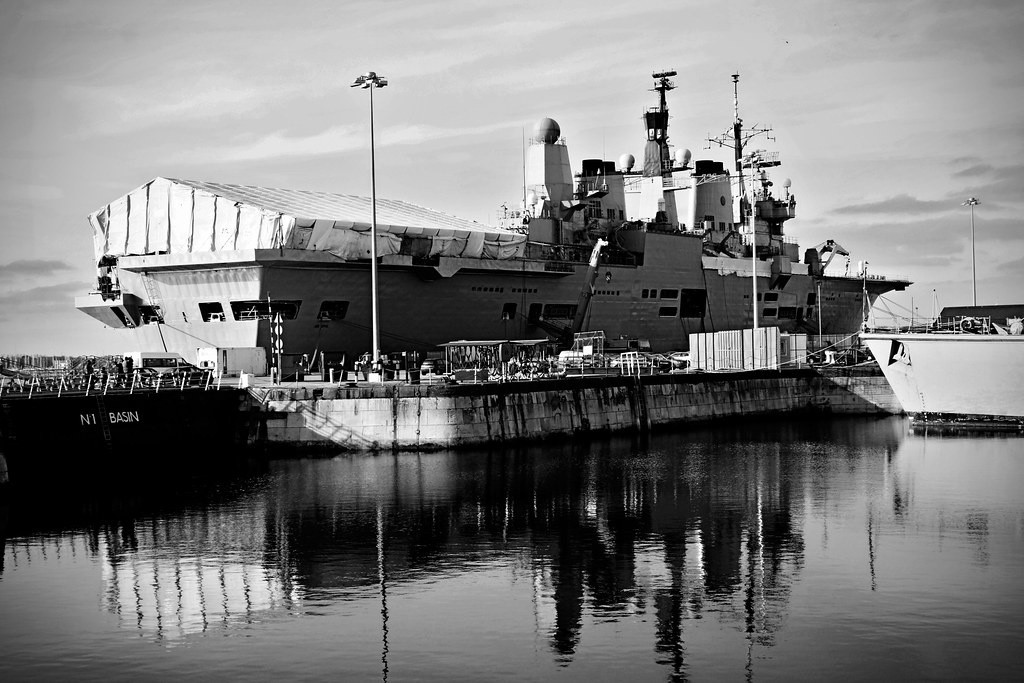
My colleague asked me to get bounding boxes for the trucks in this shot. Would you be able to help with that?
[420,358,452,376]
[193,346,272,376]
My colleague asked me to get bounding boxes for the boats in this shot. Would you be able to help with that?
[856,261,1023,437]
[70,69,911,378]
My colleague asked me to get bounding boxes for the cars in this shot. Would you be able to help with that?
[609,350,691,374]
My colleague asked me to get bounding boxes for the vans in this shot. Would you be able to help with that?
[123,352,194,375]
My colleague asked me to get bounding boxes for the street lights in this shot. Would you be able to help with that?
[350,72,389,382]
[742,151,767,330]
[961,198,988,307]
